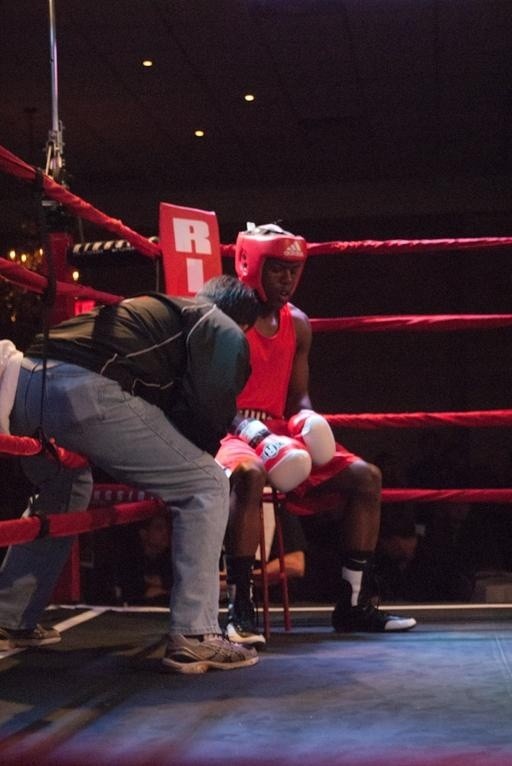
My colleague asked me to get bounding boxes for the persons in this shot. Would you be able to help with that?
[0,484,512,611]
[2,273,260,677]
[199,217,416,643]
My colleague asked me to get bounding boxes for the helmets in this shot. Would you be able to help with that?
[233,223,309,308]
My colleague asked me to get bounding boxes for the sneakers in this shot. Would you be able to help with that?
[0,623,63,652]
[162,632,261,676]
[223,602,267,646]
[332,600,418,633]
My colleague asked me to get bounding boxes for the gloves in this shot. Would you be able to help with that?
[287,408,336,466]
[234,417,313,494]
